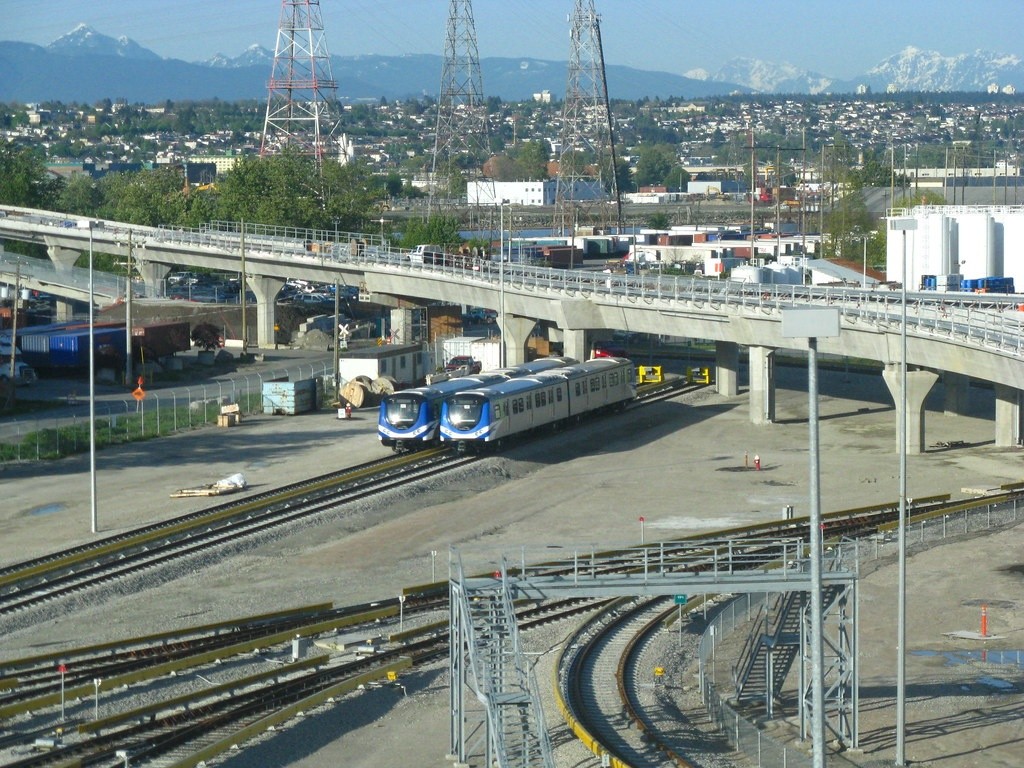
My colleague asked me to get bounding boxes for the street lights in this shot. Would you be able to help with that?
[889,217,918,767]
[500,198,512,369]
[76,220,106,534]
[781,306,841,768]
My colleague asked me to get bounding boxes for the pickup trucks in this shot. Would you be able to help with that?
[405,245,449,267]
[445,355,482,375]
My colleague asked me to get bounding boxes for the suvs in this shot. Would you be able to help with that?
[168,272,194,287]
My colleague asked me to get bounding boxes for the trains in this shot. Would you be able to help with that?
[377,357,579,455]
[439,357,636,456]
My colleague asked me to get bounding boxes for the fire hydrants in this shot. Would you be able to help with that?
[753,455,761,471]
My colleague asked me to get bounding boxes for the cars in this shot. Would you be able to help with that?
[279,280,359,304]
[183,278,246,303]
